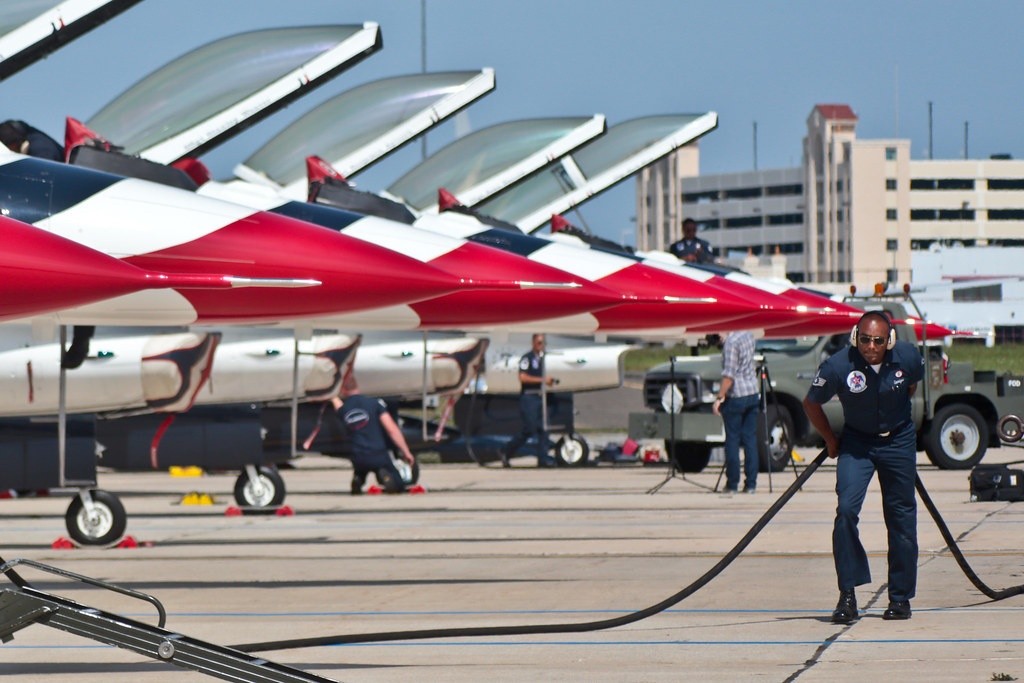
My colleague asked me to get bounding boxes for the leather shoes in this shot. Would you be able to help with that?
[883,599,911,620]
[831,588,858,623]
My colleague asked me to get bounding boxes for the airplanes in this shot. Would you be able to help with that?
[0,0,994,550]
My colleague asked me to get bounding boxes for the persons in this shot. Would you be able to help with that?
[498,333,557,468]
[713,321,761,496]
[333,374,415,493]
[804,309,923,624]
[669,217,711,265]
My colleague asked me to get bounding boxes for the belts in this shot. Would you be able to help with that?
[876,431,892,438]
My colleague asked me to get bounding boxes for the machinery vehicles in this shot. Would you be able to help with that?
[623,287,1024,473]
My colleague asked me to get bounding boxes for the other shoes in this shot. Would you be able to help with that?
[351,477,362,494]
[742,488,754,493]
[723,487,736,491]
[538,459,556,468]
[498,449,510,467]
[384,476,396,494]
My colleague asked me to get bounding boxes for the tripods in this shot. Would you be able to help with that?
[644,355,721,495]
[713,356,802,494]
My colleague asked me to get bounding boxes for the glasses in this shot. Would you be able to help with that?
[535,341,545,346]
[857,333,889,344]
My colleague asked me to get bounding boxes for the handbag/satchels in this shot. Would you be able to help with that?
[968,466,1024,503]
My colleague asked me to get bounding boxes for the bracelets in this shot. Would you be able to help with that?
[717,395,725,401]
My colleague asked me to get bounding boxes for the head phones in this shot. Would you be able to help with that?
[848,311,896,351]
